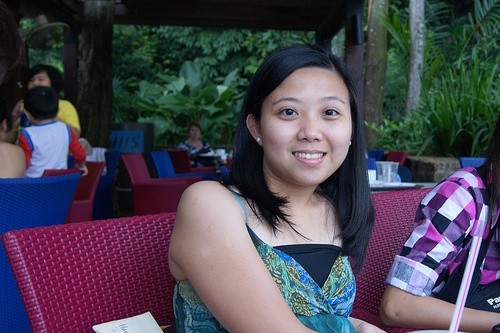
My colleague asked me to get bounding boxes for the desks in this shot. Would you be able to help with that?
[370,183,425,192]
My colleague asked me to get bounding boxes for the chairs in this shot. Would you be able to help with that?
[0,151,487,333]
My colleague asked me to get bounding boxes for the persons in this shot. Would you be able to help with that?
[178,122,214,168]
[0,64,96,177]
[380,112,500,333]
[167,44,388,333]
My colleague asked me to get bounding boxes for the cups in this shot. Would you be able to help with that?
[375,162,399,182]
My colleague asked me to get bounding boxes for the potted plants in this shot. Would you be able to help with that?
[362,114,425,165]
